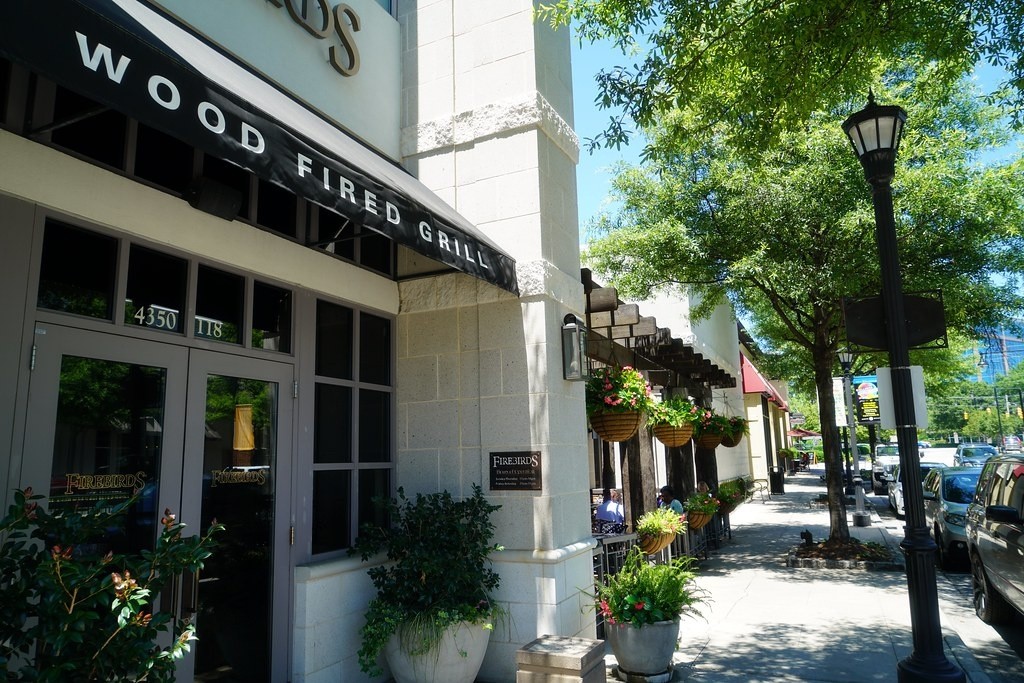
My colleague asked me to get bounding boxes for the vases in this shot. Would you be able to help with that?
[654,423,699,448]
[641,533,677,554]
[719,494,749,513]
[687,512,716,529]
[604,617,682,683]
[696,430,741,450]
[589,409,641,441]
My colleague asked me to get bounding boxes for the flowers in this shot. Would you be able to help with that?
[682,492,721,516]
[645,397,699,432]
[574,545,715,651]
[692,406,750,438]
[716,478,745,501]
[635,506,689,537]
[584,364,657,419]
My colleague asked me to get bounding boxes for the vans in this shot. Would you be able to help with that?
[871,444,925,495]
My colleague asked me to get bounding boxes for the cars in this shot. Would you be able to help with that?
[843,443,872,472]
[918,441,932,448]
[885,463,948,519]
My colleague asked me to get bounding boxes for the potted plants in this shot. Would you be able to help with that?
[346,486,517,683]
[778,448,790,458]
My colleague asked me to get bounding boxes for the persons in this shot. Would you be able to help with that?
[594,481,710,523]
[800,452,810,472]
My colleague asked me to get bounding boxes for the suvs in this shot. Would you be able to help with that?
[922,465,985,565]
[953,444,999,467]
[1000,436,1023,453]
[967,453,1024,623]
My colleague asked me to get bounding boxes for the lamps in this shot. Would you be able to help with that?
[232,401,255,451]
[561,326,582,380]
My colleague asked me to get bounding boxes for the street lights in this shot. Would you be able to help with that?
[837,346,871,527]
[844,85,966,683]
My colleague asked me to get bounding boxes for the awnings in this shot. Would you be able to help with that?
[739,349,796,415]
[2,0,521,299]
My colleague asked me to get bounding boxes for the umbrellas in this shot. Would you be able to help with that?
[785,427,822,466]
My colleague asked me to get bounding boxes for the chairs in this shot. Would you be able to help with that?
[742,475,771,505]
